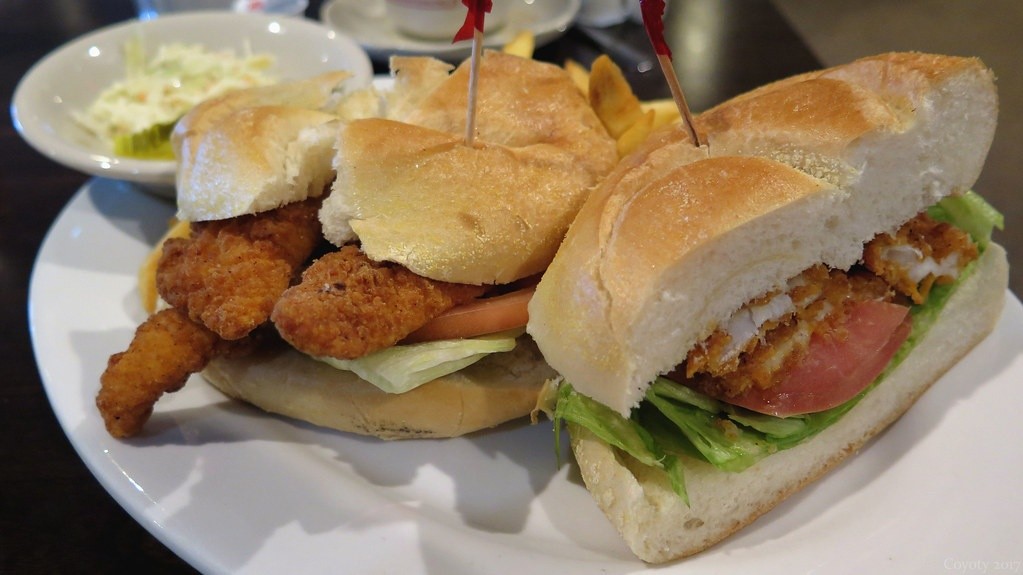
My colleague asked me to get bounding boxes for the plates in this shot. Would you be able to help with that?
[26,76,1023,575]
[11,11,373,183]
[321,0,582,59]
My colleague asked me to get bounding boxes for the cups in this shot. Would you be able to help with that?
[385,1,505,38]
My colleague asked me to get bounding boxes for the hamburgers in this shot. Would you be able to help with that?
[91,53,618,443]
[510,47,1010,564]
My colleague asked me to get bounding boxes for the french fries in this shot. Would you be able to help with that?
[500,34,702,159]
